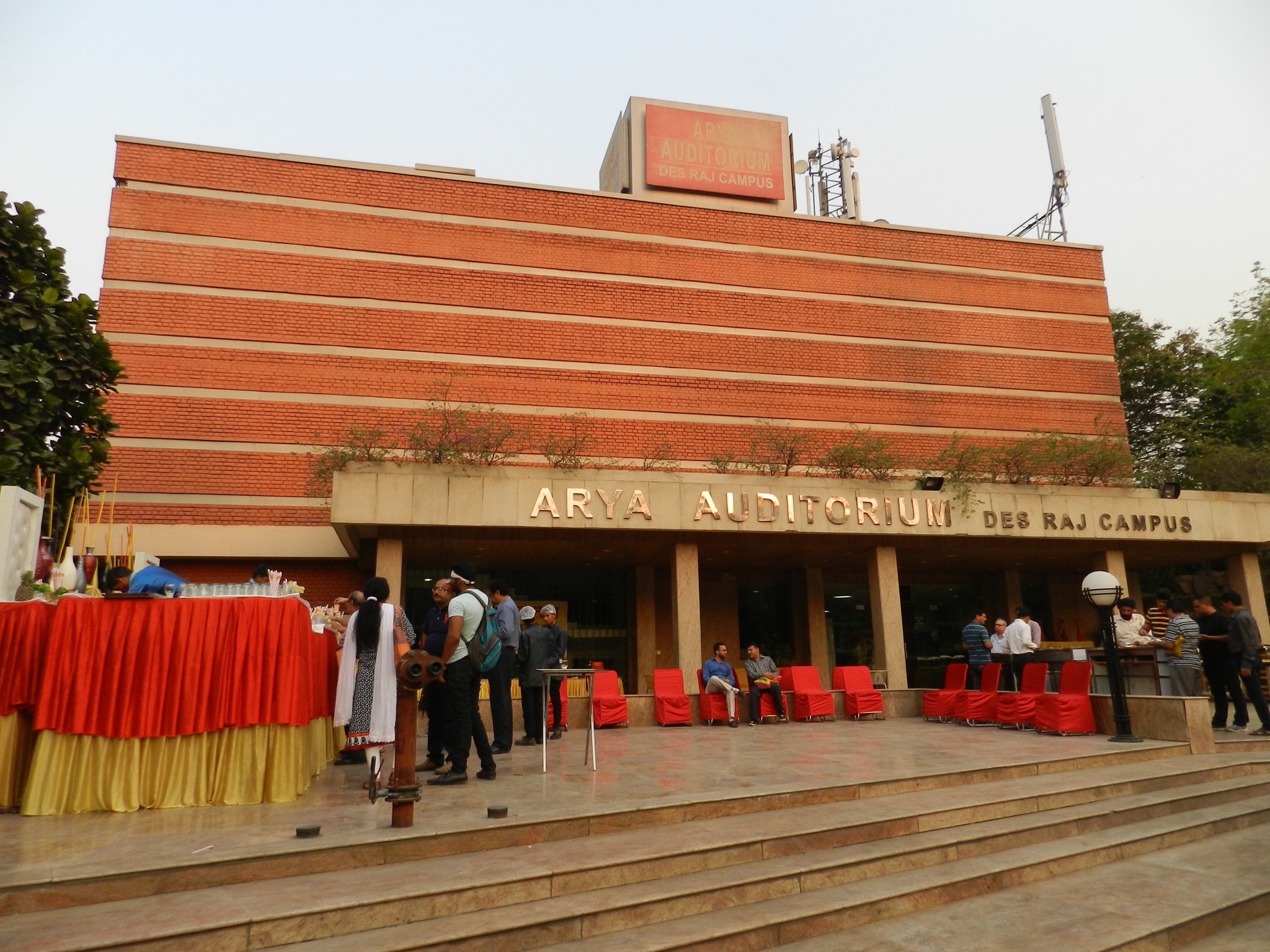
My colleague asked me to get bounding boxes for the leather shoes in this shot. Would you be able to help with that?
[514,736,536,746]
[729,721,738,728]
[435,761,453,775]
[536,737,543,744]
[476,770,497,780]
[490,745,509,754]
[427,770,468,785]
[550,731,562,740]
[1249,727,1270,735]
[415,759,445,771]
[738,690,746,698]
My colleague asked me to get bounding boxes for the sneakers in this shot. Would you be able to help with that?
[779,710,786,721]
[749,721,757,726]
[1212,724,1227,731]
[1226,724,1247,732]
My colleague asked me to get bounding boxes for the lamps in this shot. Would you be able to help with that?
[919,475,944,491]
[1158,481,1181,499]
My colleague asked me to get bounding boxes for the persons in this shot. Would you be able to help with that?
[414,578,454,774]
[334,577,416,789]
[244,567,273,585]
[105,565,190,598]
[330,590,367,764]
[539,604,566,739]
[961,605,1041,693]
[1101,585,1270,736]
[702,642,746,727]
[481,578,522,755]
[514,606,557,745]
[429,563,497,787]
[744,643,786,726]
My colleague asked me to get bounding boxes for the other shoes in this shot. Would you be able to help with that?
[363,781,381,790]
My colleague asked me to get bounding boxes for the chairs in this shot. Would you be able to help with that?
[955,663,1002,725]
[997,663,1049,732]
[842,666,885,721]
[546,675,569,732]
[923,662,968,721]
[745,671,790,725]
[791,665,837,723]
[832,667,846,691]
[697,668,739,726]
[590,670,629,729]
[1035,661,1097,736]
[652,668,693,727]
[777,667,795,690]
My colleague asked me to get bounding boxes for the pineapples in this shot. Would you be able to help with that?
[15,571,39,602]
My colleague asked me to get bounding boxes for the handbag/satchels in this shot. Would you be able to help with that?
[754,675,782,689]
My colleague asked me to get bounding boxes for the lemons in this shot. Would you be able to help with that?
[289,581,306,594]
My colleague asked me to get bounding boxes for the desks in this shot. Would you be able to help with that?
[415,675,626,696]
[307,618,346,776]
[1036,644,1095,695]
[1085,642,1172,697]
[15,595,318,804]
[0,601,55,810]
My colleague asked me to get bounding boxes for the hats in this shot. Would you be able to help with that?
[1155,588,1172,601]
[1117,597,1136,609]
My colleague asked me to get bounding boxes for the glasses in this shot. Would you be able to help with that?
[994,624,1005,627]
[432,587,452,593]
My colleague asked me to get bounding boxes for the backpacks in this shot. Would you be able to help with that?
[460,590,502,674]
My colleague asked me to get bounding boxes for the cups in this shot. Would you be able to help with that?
[309,611,340,624]
[165,584,175,598]
[179,584,298,596]
